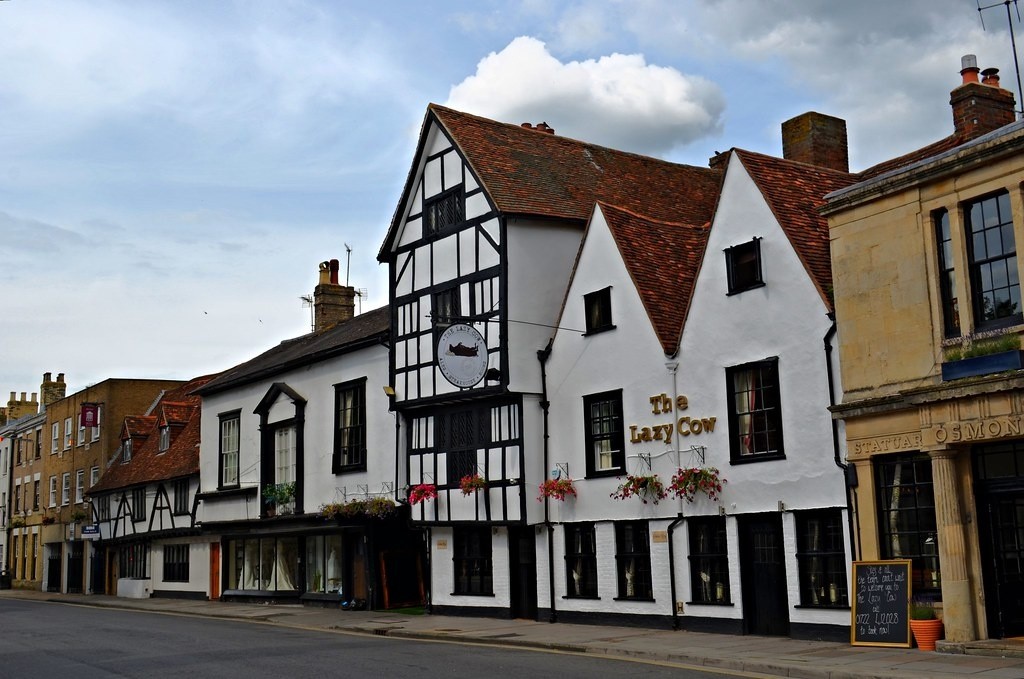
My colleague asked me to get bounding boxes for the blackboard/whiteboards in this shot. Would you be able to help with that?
[851,558,913,648]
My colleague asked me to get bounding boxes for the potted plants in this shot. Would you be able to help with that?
[261,481,298,518]
[940,330,1024,382]
[909,598,942,651]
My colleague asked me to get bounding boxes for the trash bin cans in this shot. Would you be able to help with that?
[0,570,11,590]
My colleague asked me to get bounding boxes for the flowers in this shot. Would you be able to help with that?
[535,478,577,503]
[317,497,396,520]
[609,472,665,506]
[459,474,486,498]
[409,484,438,506]
[667,468,727,505]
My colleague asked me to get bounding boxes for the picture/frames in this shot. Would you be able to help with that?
[850,559,913,648]
